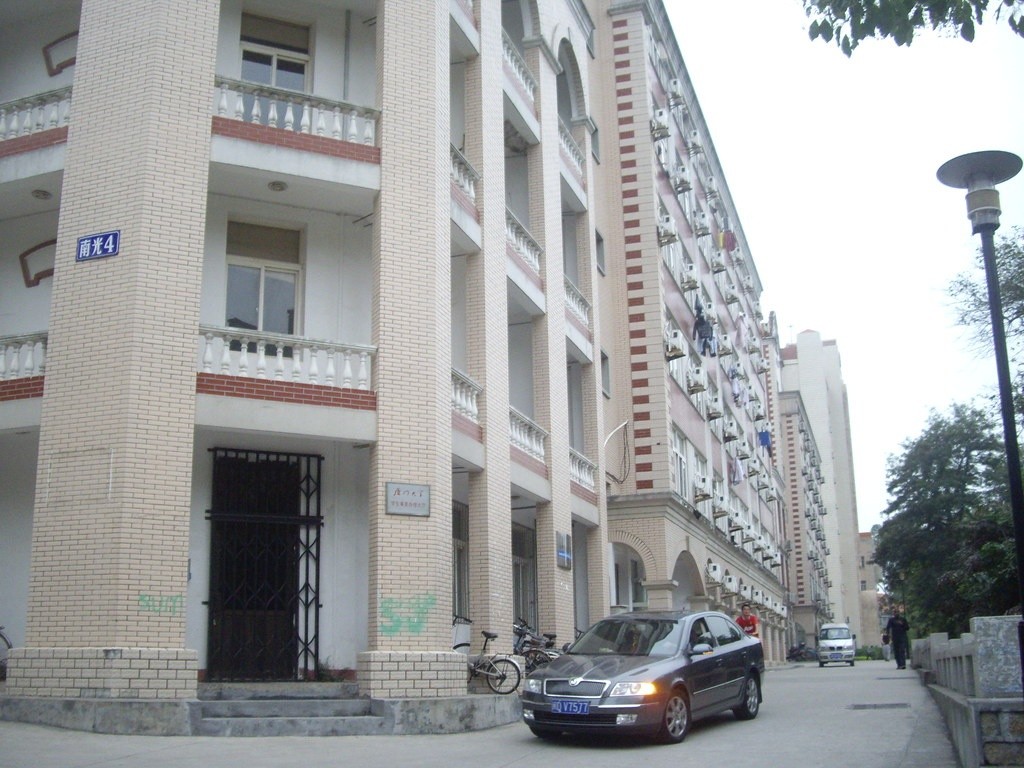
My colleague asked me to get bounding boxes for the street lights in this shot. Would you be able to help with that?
[937,148,1024,690]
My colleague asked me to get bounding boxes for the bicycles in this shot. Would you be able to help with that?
[514,615,587,678]
[450,612,522,695]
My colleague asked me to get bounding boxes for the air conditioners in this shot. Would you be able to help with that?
[695,476,782,567]
[704,563,787,619]
[652,79,777,502]
[800,419,835,619]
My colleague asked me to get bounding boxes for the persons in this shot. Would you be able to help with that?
[866,645,874,660]
[735,604,759,638]
[881,627,890,661]
[886,608,910,669]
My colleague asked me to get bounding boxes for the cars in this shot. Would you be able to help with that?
[519,608,767,744]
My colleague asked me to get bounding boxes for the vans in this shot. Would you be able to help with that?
[815,623,857,668]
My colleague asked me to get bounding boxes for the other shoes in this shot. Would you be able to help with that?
[897,665,906,670]
